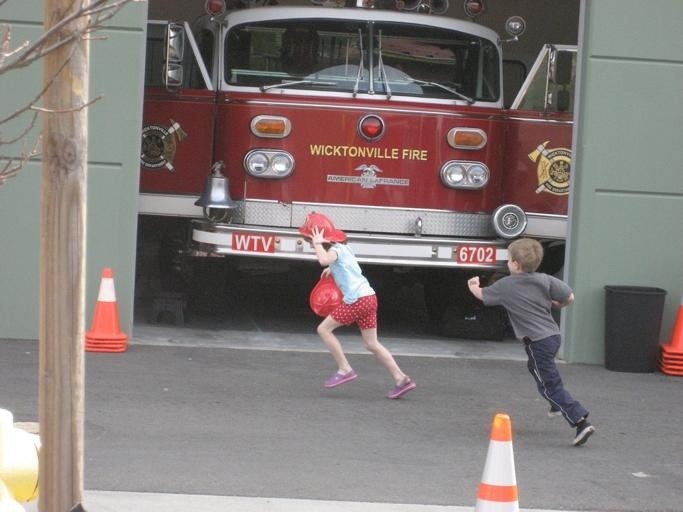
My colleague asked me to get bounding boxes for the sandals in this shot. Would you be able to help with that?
[324,368,358,388]
[386,376,416,399]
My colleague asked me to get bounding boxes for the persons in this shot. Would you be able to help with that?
[466,238,595,447]
[300,212,417,400]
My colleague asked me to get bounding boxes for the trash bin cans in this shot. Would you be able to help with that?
[605,286,666,373]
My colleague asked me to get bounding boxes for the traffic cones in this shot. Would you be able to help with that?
[658,297,683,376]
[84,266,128,353]
[473,414,520,512]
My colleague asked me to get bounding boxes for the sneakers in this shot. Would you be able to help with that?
[572,422,595,446]
[547,407,562,417]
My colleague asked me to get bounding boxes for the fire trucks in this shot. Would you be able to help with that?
[138,0,578,338]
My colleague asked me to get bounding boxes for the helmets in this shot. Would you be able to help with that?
[298,213,348,243]
[309,273,343,318]
